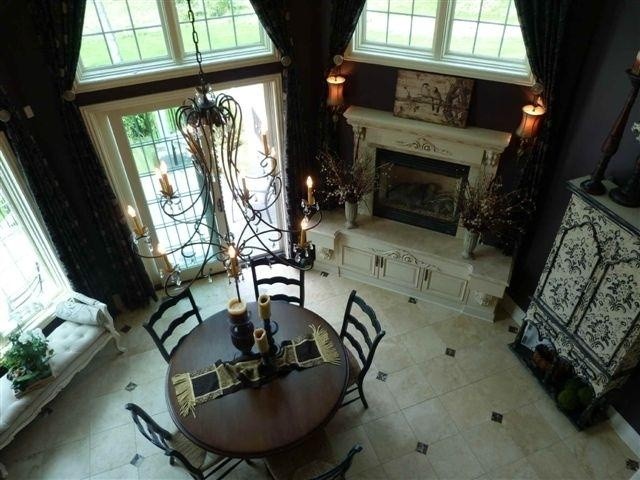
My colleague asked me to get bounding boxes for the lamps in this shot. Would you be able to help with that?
[124,0,324,303]
[326,64,346,123]
[516,94,545,157]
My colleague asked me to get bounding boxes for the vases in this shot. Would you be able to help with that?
[7,357,56,399]
[462,231,480,260]
[345,202,358,229]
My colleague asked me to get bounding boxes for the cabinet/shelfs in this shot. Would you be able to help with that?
[508,172,639,432]
[421,269,468,312]
[342,246,424,300]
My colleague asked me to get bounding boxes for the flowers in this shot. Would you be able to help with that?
[0,326,57,376]
[312,143,399,225]
[424,164,540,253]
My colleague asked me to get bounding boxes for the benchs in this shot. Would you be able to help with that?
[0,289,126,479]
